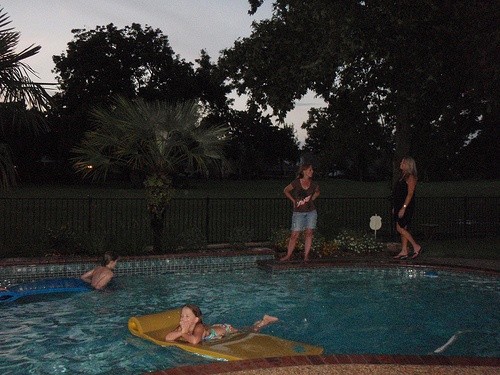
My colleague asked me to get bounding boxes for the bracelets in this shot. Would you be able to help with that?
[403,204,407,208]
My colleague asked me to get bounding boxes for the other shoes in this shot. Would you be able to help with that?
[393,254,408,260]
[412,247,423,259]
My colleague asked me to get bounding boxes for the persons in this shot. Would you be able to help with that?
[391,156,423,259]
[81,252,118,289]
[280,163,320,261]
[166,304,279,344]
